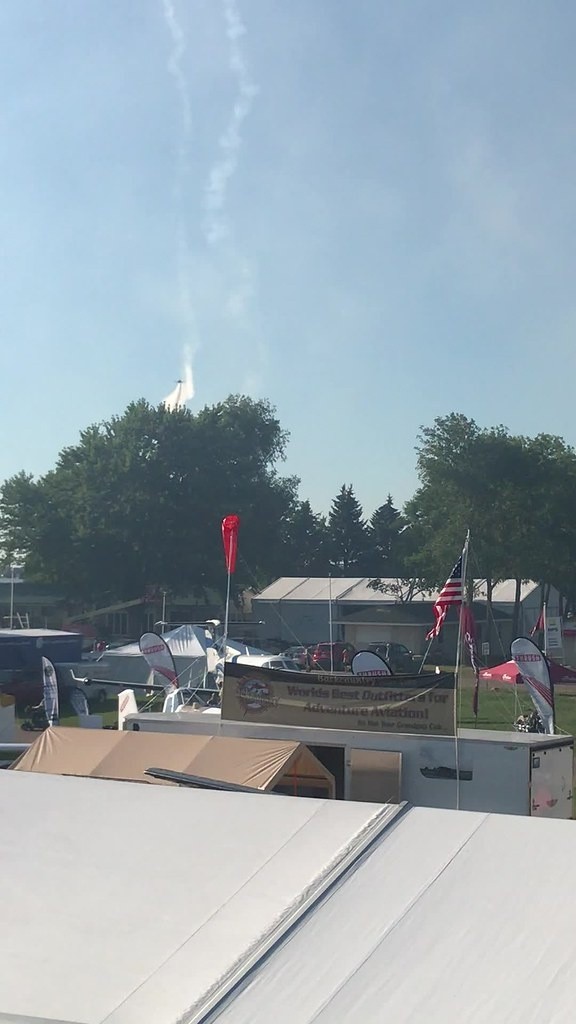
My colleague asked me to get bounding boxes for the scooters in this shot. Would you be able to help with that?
[19,703,50,732]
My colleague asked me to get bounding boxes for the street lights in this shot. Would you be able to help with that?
[8,565,23,629]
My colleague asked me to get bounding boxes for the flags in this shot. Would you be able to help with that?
[425,547,468,640]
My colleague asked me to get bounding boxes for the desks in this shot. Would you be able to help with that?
[176,704,221,714]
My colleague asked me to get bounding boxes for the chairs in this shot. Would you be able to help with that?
[22,704,49,730]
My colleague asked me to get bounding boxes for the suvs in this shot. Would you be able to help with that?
[310,639,359,672]
[368,641,423,674]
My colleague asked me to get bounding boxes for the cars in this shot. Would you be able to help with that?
[282,645,308,666]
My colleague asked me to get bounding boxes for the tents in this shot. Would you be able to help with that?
[7,726,336,801]
[106,623,275,690]
